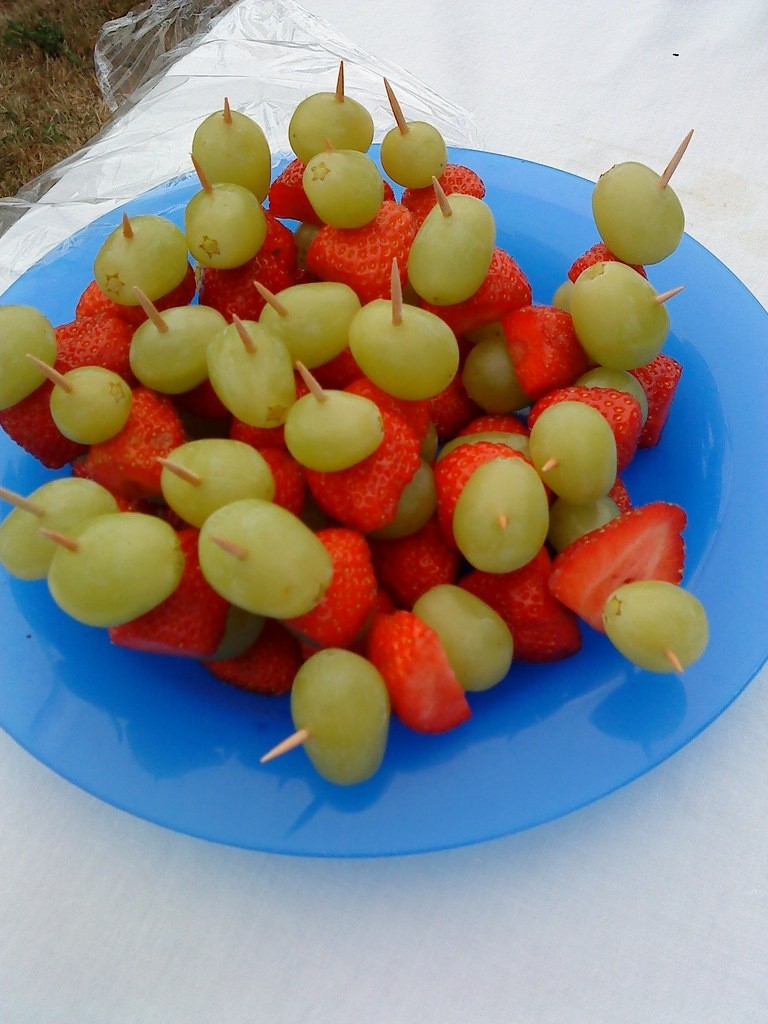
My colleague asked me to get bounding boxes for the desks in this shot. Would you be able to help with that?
[0,0,768,1024]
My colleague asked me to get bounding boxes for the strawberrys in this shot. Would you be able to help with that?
[0,160,686,731]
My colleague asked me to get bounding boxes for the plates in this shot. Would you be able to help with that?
[0,144,768,855]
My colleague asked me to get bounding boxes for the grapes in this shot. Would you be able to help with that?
[0,91,712,782]
